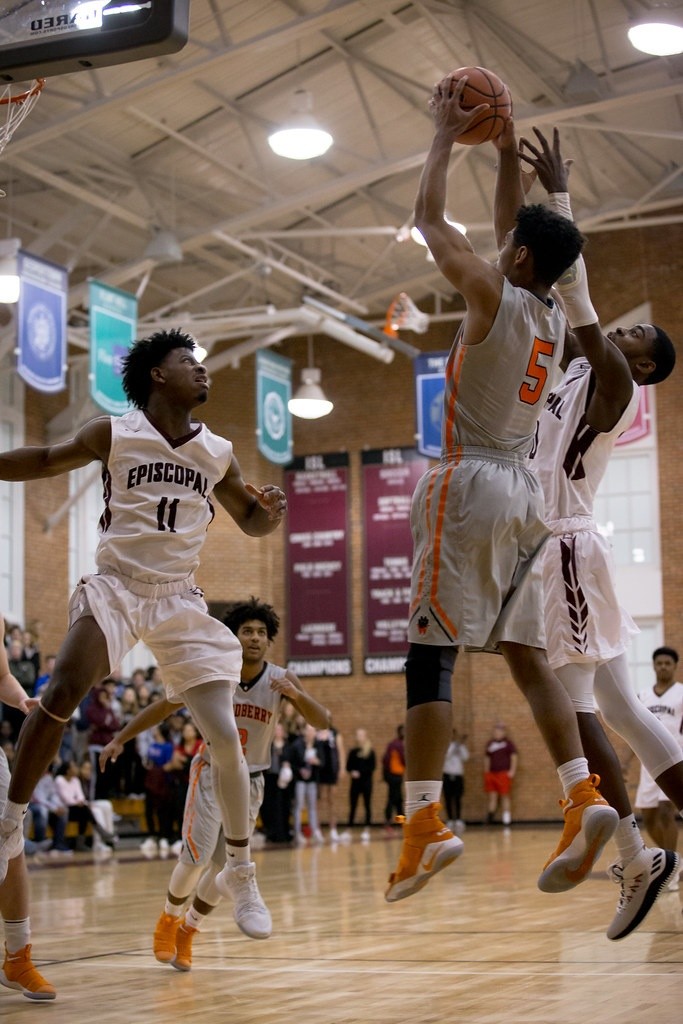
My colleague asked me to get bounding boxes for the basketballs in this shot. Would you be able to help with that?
[437,65,513,146]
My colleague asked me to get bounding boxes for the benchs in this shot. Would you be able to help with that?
[27,797,150,842]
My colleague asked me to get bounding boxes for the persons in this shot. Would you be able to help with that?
[0,329,289,942]
[383,70,618,902]
[442,727,471,831]
[620,646,683,891]
[338,728,377,840]
[1,612,59,998]
[484,721,518,826]
[21,663,205,861]
[0,617,57,775]
[513,123,682,941]
[98,594,333,971]
[256,702,347,846]
[381,723,404,831]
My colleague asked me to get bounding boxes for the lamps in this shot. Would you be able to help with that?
[269,84,334,160]
[288,337,333,420]
[409,209,466,245]
[145,229,183,264]
[628,8,683,56]
[0,241,22,303]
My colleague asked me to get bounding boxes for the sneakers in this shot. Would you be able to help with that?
[172,907,198,972]
[607,848,677,940]
[153,912,181,962]
[0,942,56,1000]
[539,773,619,893]
[384,802,465,902]
[215,860,271,938]
